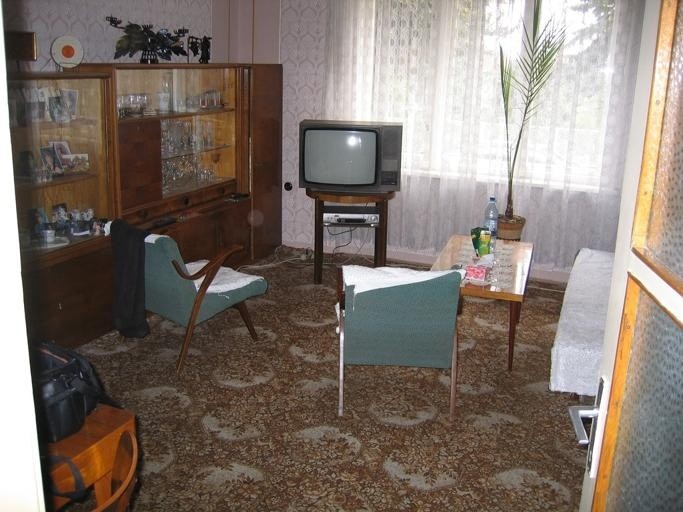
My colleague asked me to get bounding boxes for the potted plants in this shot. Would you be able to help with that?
[105,15,190,62]
[497,0,566,241]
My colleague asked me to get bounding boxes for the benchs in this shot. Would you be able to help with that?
[548,248,618,404]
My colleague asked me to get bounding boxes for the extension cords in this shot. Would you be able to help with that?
[300,253,314,261]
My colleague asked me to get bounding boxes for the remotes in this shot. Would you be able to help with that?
[450,264,462,270]
[154,216,176,227]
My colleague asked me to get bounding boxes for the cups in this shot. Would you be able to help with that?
[116,89,225,193]
[40,229,56,243]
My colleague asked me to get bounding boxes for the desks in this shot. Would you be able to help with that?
[306,186,396,284]
[44,402,136,512]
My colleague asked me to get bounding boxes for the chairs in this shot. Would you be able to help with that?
[89,431,139,512]
[111,220,269,372]
[335,264,461,422]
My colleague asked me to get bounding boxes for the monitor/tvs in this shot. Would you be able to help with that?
[298,119,403,193]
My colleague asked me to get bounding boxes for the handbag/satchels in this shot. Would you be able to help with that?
[30,341,102,443]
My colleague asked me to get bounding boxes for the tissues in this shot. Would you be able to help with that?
[463,253,495,280]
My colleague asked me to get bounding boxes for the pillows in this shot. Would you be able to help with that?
[340,263,468,298]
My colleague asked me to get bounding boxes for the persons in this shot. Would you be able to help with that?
[42,149,61,174]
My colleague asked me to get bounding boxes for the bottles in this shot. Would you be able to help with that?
[483,196,499,251]
